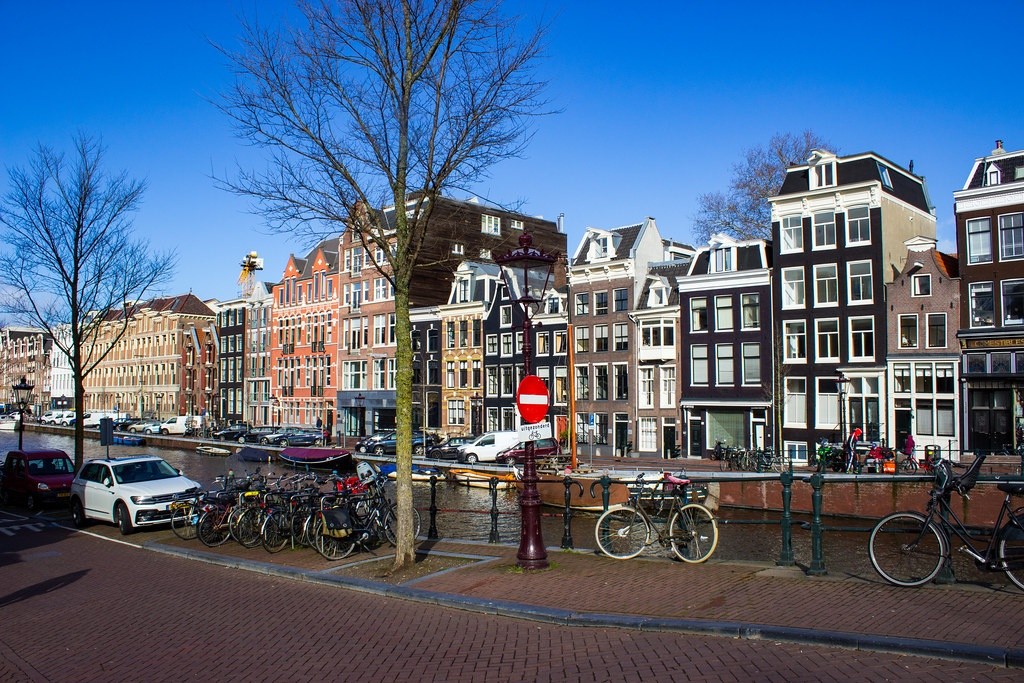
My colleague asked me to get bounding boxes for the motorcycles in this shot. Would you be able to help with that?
[814,438,847,473]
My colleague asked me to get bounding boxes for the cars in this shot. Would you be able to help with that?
[496,437,564,466]
[3,449,79,511]
[426,437,477,460]
[367,431,435,457]
[211,424,321,446]
[10,411,26,421]
[355,430,393,454]
[37,408,163,435]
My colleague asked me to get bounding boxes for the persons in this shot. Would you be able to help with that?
[867,443,883,472]
[883,449,895,462]
[316,417,322,427]
[846,428,862,474]
[905,435,915,469]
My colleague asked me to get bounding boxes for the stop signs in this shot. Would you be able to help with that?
[516,375,549,423]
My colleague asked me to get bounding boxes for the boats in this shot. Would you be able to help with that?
[194,445,230,456]
[280,446,352,472]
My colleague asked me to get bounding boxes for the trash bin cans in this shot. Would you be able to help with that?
[925,445,941,470]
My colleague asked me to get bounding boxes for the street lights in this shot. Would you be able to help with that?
[356,392,365,443]
[494,232,558,569]
[9,376,35,451]
[61,394,67,414]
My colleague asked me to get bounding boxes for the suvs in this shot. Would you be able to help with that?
[71,452,203,535]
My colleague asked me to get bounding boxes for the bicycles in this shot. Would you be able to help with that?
[712,438,790,474]
[868,453,1024,590]
[593,471,720,565]
[899,445,934,474]
[169,464,422,561]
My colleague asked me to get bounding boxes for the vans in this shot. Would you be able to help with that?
[455,432,524,465]
[160,415,203,436]
